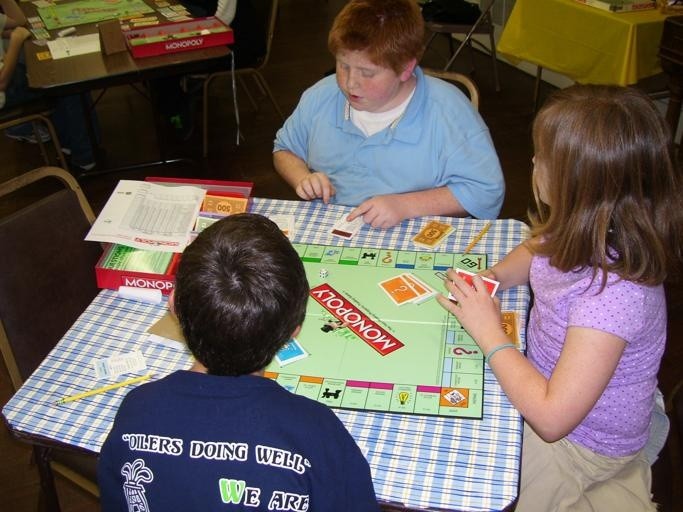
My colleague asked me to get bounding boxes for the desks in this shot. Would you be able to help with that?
[18,0,233,177]
[494,1,683,159]
[1,196,532,512]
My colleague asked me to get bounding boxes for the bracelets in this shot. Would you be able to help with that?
[485,344,517,363]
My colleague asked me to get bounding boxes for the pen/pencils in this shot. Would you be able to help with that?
[462,223,492,257]
[53,373,159,405]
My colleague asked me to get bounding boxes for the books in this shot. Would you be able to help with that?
[579,0,656,12]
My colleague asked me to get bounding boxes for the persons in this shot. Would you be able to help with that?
[180,0,273,74]
[435,87,682,512]
[272,0,507,231]
[97,214,378,511]
[0,0,97,170]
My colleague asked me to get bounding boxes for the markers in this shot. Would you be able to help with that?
[57,27,75,37]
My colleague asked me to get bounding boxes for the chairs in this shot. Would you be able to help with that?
[422,1,494,71]
[181,0,286,158]
[652,380,683,511]
[422,69,479,116]
[0,97,70,173]
[1,166,106,512]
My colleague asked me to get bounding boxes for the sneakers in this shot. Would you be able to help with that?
[171,101,193,143]
[6,125,96,182]
